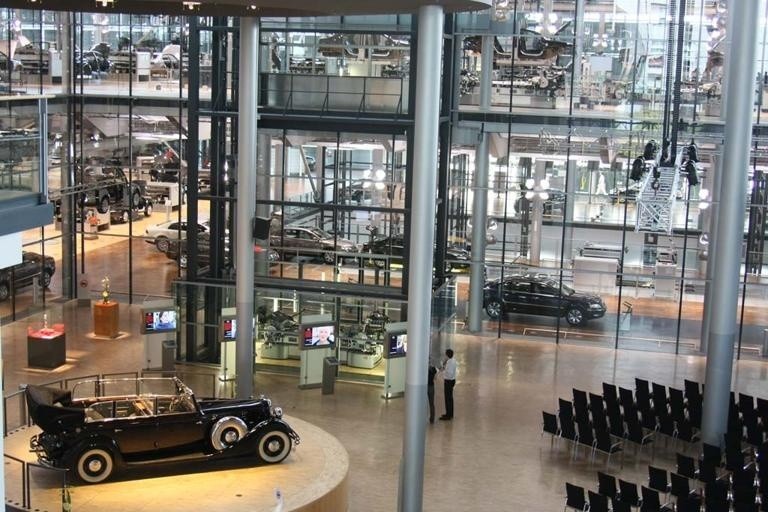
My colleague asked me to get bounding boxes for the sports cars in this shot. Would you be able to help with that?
[23,376,300,486]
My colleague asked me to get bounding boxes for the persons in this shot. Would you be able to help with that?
[428,356,437,424]
[305,328,312,337]
[88,212,98,226]
[146,313,153,323]
[165,148,173,159]
[397,335,407,353]
[703,67,712,81]
[668,237,678,264]
[313,327,334,345]
[439,349,458,420]
[691,68,696,87]
[155,311,174,328]
[225,321,231,330]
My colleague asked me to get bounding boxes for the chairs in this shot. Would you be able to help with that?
[538,378,768,512]
[54,395,162,423]
[507,281,542,294]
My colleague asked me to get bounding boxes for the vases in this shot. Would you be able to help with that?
[104,298,109,304]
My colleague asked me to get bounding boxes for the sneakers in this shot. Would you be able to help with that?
[439,413,454,421]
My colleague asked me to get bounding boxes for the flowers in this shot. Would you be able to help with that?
[101,276,111,298]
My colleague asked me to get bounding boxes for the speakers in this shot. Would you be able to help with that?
[253,217,272,240]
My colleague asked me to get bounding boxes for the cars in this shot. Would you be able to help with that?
[338,177,396,202]
[462,28,573,67]
[610,182,684,203]
[165,229,280,267]
[144,218,210,252]
[270,225,358,265]
[513,188,567,214]
[0,250,55,302]
[0,41,180,82]
[317,31,411,60]
[483,274,606,326]
[45,151,229,228]
[362,233,470,273]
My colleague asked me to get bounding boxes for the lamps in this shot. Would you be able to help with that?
[629,140,701,186]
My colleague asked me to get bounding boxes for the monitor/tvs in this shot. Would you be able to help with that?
[386,331,407,359]
[301,322,338,350]
[220,314,258,341]
[141,306,179,333]
